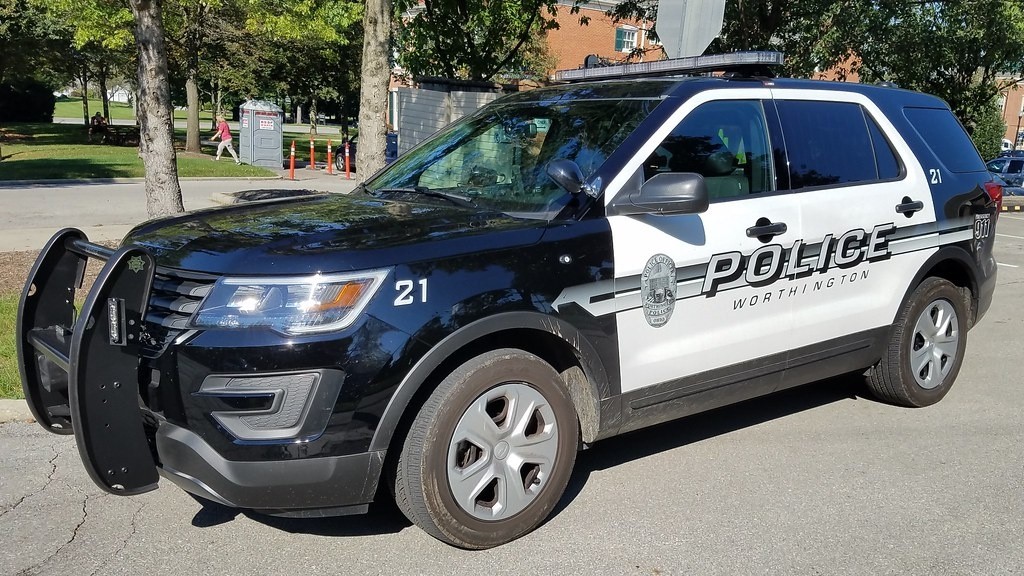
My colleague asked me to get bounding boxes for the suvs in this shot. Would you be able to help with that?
[16,52,1004,551]
[997,149,1024,158]
[335,134,397,171]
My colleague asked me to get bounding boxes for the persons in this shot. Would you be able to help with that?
[89,112,107,144]
[211,116,241,165]
[309,115,318,134]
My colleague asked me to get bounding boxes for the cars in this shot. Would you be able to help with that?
[985,157,1024,196]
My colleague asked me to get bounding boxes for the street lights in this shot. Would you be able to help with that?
[1012,112,1023,150]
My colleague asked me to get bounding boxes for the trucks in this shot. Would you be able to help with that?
[1001,138,1016,150]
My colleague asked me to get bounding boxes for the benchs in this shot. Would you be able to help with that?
[91,125,141,146]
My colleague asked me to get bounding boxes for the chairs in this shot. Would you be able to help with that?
[667,143,750,200]
